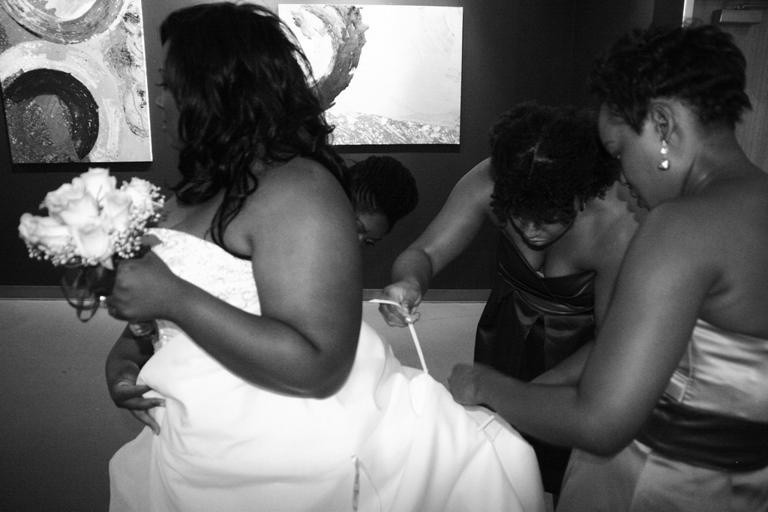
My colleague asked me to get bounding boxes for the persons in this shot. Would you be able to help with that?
[349,156,419,247]
[446,17,767,512]
[106,2,545,512]
[380,100,649,512]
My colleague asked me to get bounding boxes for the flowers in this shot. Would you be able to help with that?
[17,167,169,338]
